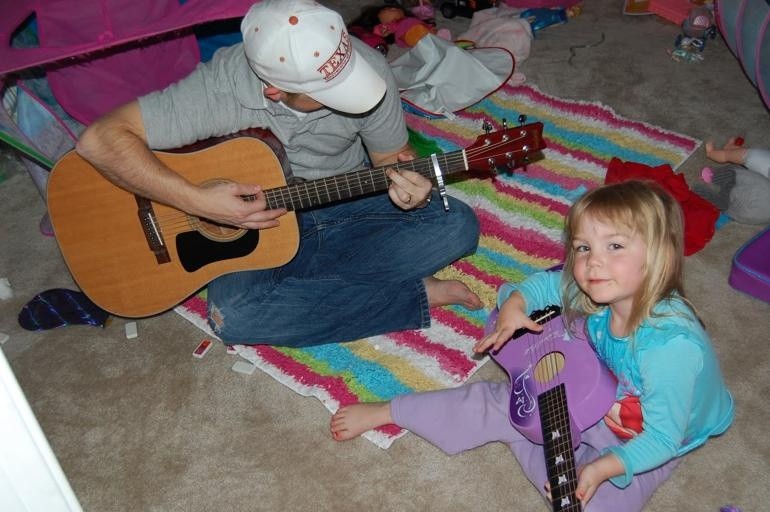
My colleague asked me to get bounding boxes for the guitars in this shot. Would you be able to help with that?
[46,116,547,321]
[484,298,619,511]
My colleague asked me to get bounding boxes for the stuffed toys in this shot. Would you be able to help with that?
[674,7,716,52]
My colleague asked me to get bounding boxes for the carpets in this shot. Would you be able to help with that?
[171,81,704,450]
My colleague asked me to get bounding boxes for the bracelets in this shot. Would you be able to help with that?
[417,193,432,209]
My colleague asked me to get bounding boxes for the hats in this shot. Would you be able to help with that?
[240,0,387,114]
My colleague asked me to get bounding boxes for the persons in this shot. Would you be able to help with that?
[329,178,738,511]
[73,0,486,349]
[371,6,453,49]
[500,5,583,33]
[704,135,770,184]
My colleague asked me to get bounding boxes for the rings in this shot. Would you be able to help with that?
[405,197,411,203]
